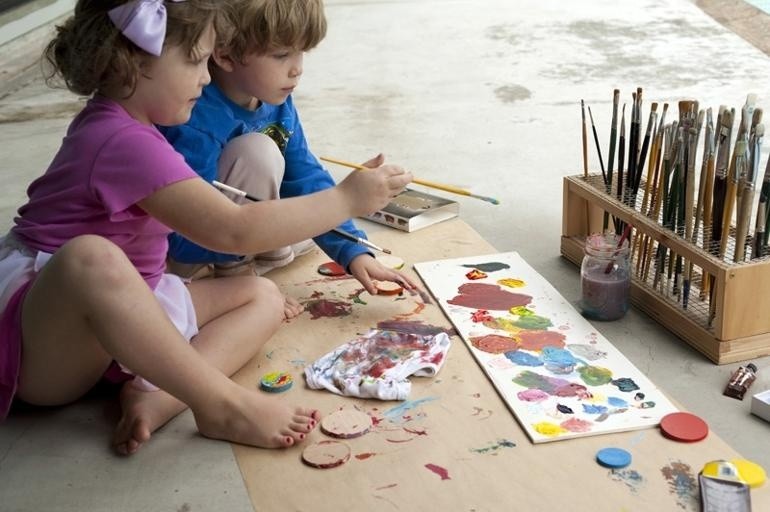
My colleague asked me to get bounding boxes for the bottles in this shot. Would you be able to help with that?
[576,231,633,320]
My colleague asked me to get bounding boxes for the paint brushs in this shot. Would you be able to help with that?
[581,87,770,327]
[211,179,392,256]
[319,156,498,206]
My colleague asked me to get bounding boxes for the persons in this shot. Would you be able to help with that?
[152,1,417,321]
[0,1,415,459]
